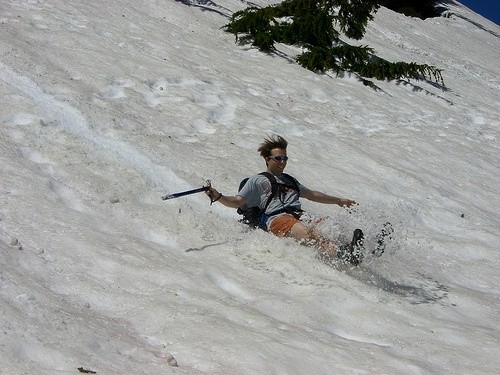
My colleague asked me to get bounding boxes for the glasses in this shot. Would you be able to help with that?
[268,156,288,161]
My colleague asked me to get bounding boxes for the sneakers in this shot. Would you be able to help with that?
[373,221,394,257]
[337,229,365,266]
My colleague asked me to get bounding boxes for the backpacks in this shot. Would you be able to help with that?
[236,172,300,228]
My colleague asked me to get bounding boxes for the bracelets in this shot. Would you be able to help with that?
[211,193,222,203]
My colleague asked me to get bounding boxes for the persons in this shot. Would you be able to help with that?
[207,138,395,267]
